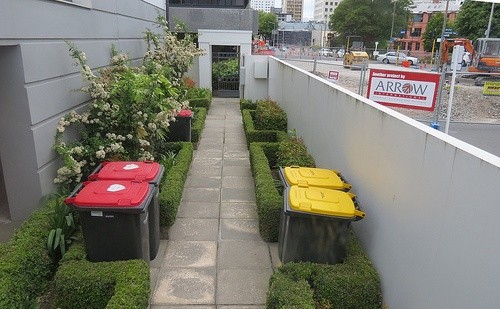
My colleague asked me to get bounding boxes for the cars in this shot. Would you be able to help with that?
[337,48,346,58]
[376,52,417,65]
[318,48,332,57]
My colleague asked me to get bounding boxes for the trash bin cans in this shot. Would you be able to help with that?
[276,184,365,264]
[88,161,166,261]
[168,108,194,142]
[277,165,353,262]
[63,181,155,262]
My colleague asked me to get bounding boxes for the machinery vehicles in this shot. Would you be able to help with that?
[439,37,500,78]
[342,51,368,70]
